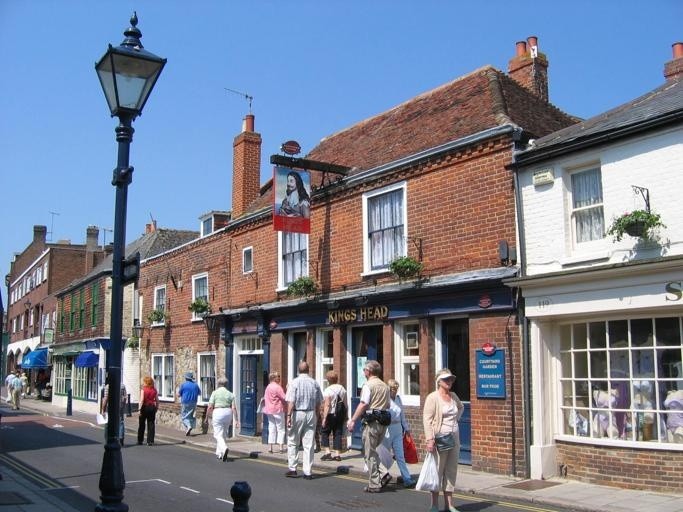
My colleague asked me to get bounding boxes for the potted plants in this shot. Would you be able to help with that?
[607,210,668,243]
[287,276,316,299]
[128,336,139,350]
[389,256,424,281]
[185,297,211,314]
[147,311,165,323]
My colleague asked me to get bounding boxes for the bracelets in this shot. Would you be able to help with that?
[287,414,291,417]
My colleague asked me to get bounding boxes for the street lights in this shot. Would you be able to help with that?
[95,11,167,512]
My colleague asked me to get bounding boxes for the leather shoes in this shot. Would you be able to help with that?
[445,505,460,512]
[303,474,313,480]
[286,471,297,478]
[430,505,439,512]
[222,447,229,462]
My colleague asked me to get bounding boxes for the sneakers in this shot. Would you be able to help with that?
[404,478,417,487]
[333,456,341,461]
[381,473,392,488]
[320,453,332,461]
[185,426,192,436]
[363,486,382,493]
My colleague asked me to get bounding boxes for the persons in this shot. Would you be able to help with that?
[320,373,348,461]
[136,376,159,446]
[279,171,310,220]
[5,371,29,410]
[284,362,324,480]
[101,383,127,447]
[203,377,239,462]
[265,372,289,454]
[177,373,201,435]
[381,378,417,488]
[347,360,390,493]
[422,366,464,512]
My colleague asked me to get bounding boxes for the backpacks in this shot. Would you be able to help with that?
[328,386,347,420]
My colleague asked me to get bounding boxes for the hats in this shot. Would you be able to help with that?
[436,372,457,383]
[184,372,195,380]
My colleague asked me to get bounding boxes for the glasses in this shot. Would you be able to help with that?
[442,377,453,382]
[363,367,368,371]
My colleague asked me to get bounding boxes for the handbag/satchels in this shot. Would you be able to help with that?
[403,430,418,464]
[377,410,392,426]
[435,431,455,452]
[256,397,265,414]
